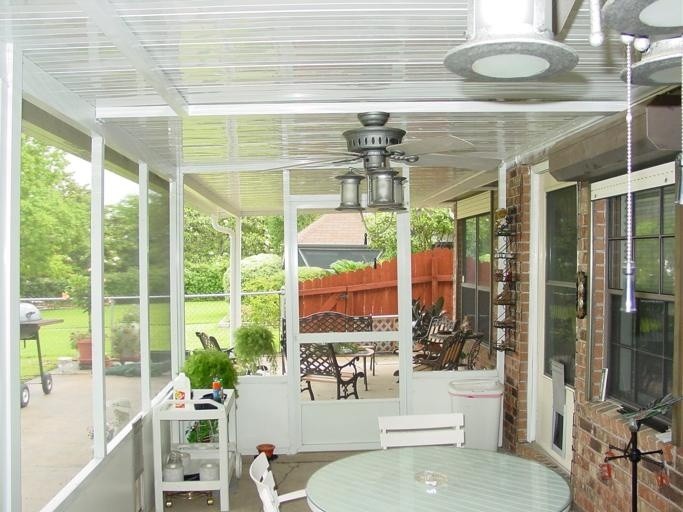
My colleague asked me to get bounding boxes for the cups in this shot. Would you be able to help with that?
[164,453,220,482]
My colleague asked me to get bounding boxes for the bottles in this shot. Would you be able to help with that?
[172,371,191,410]
[213,380,223,403]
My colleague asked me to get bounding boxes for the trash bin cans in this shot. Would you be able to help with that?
[447,378,506,452]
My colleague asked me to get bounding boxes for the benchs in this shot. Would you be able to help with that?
[279,311,378,375]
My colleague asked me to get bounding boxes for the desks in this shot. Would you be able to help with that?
[305,446,572,512]
[21,318,64,408]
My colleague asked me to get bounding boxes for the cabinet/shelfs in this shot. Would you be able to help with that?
[149,380,242,511]
[491,205,517,352]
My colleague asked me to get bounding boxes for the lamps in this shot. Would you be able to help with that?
[444,0,682,88]
[332,155,407,212]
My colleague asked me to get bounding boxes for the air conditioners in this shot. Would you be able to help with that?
[548,93,681,201]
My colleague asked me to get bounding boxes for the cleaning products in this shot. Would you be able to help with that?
[173,372,190,408]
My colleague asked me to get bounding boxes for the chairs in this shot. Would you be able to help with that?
[300,343,368,400]
[248,412,464,512]
[195,330,269,380]
[392,314,483,384]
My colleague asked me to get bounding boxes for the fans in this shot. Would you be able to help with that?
[257,112,501,172]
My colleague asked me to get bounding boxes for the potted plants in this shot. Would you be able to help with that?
[68,330,108,365]
